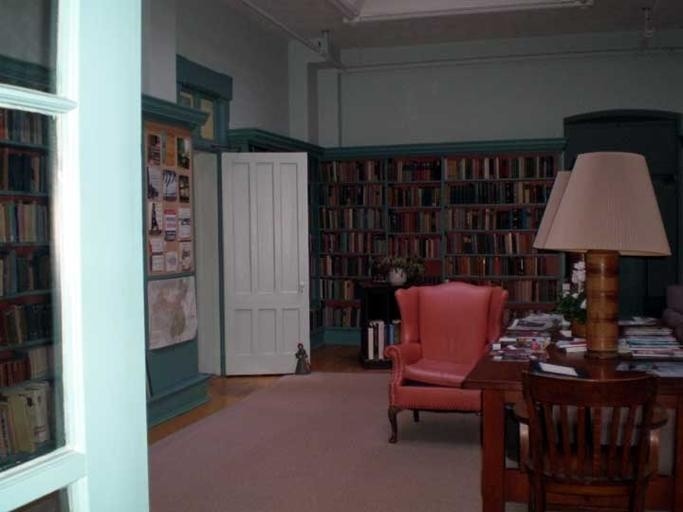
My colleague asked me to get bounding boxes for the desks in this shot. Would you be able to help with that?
[460,318,683,511]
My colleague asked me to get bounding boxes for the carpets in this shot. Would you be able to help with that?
[144,370,510,512]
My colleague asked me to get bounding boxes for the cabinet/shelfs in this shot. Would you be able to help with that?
[313,138,568,350]
[1,81,83,509]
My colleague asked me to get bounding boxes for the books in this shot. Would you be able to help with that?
[320,157,442,206]
[618,315,683,361]
[318,208,441,260]
[443,206,559,255]
[0,107,56,463]
[443,154,552,205]
[318,255,379,327]
[443,256,564,318]
[563,346,588,353]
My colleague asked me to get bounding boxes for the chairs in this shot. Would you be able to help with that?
[519,371,660,511]
[378,278,514,446]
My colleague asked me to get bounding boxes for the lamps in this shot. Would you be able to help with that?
[532,149,672,362]
